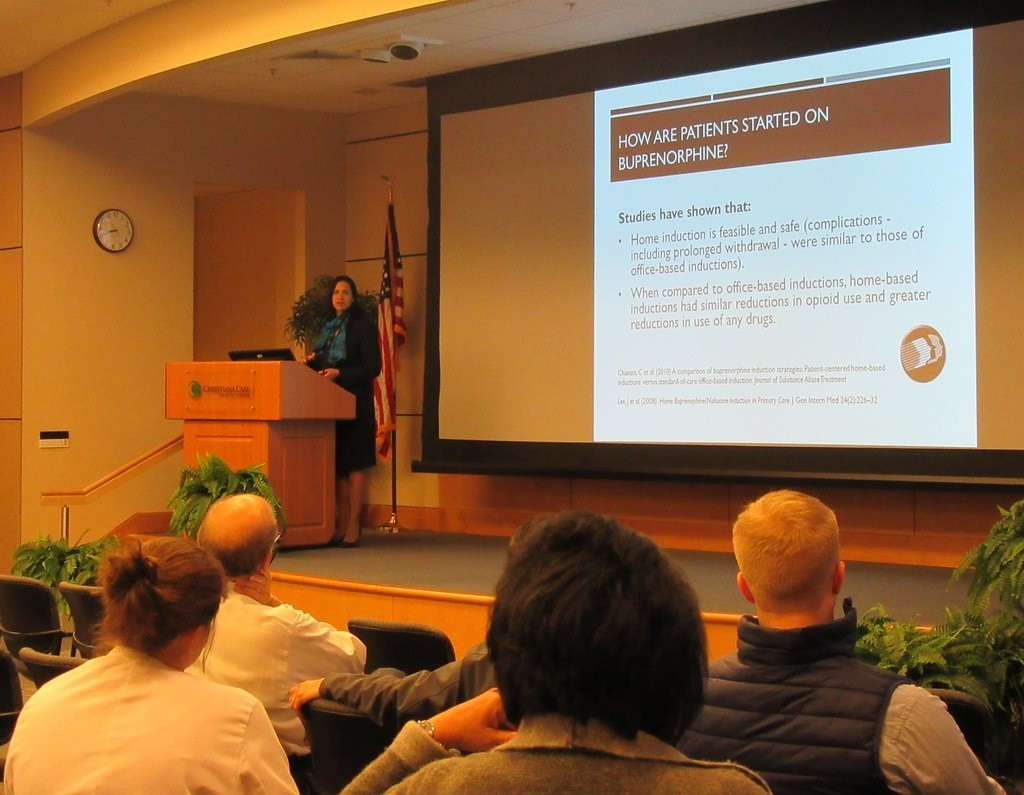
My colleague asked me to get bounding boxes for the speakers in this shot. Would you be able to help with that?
[389,42,424,61]
[362,49,390,63]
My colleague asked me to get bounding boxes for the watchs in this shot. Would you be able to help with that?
[417,721,445,748]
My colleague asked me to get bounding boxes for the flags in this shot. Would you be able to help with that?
[376,203,407,462]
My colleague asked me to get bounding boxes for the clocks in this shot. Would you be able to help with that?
[93,208,134,254]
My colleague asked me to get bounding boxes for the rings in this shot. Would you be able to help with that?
[291,697,295,702]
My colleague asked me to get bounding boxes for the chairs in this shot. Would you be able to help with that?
[349,619,456,674]
[0,576,107,747]
[300,698,398,795]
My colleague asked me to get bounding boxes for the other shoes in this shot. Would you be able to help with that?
[326,530,361,548]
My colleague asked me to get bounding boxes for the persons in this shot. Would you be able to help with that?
[301,276,383,549]
[4,537,300,795]
[675,491,1015,795]
[183,492,366,795]
[289,510,772,795]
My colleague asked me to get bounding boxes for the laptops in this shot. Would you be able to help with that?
[228,348,297,362]
